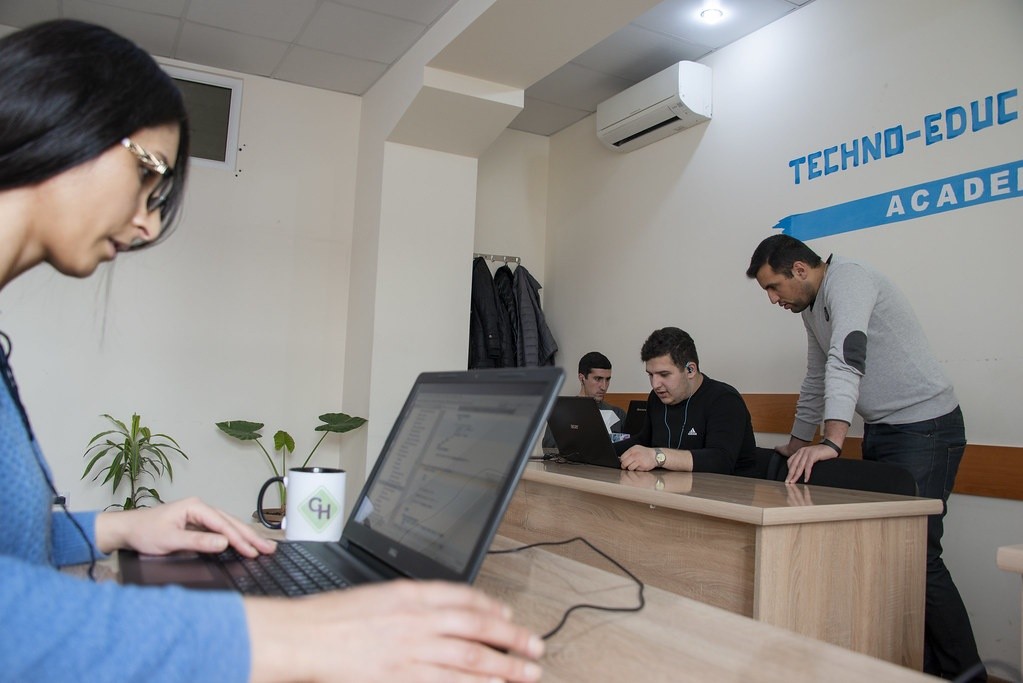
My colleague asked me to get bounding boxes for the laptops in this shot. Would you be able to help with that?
[622,400,648,435]
[546,395,628,469]
[117,367,567,599]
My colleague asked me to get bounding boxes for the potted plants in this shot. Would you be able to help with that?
[216,413,368,528]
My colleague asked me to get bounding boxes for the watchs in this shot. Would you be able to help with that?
[819,438,841,457]
[655,475,665,491]
[655,448,666,467]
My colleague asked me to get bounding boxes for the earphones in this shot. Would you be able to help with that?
[687,366,693,373]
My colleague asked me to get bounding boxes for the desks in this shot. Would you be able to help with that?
[58,526,947,683]
[498,458,942,673]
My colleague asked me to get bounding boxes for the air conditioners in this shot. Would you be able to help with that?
[596,61,713,154]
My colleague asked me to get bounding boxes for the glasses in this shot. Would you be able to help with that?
[121,137,176,213]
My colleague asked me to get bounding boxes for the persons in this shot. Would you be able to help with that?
[618,469,735,501]
[618,327,756,475]
[746,234,988,683]
[542,351,627,459]
[0,19,547,683]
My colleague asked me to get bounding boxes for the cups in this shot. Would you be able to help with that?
[256,467,347,543]
